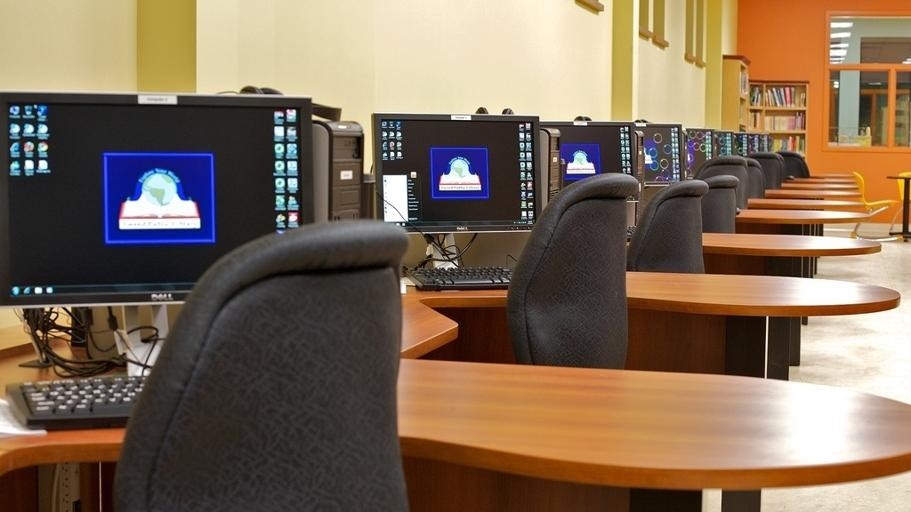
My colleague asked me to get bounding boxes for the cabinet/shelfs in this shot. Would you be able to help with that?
[748,80,808,157]
[722,55,750,133]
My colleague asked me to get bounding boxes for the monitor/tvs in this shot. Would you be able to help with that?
[715,131,734,157]
[1,91,313,346]
[734,133,747,156]
[685,128,713,177]
[759,134,768,153]
[371,113,541,266]
[636,124,681,186]
[748,133,759,154]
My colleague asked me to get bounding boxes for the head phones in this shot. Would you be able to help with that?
[475,107,515,117]
[242,86,340,121]
[635,118,647,123]
[576,116,595,122]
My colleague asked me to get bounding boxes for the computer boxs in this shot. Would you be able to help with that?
[636,131,643,217]
[313,121,364,224]
[680,131,687,181]
[541,127,559,208]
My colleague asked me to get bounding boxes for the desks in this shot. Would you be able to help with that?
[2,175,910,511]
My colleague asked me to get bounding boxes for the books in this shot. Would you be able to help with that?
[750,85,806,154]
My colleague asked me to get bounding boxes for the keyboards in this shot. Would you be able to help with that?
[406,267,512,292]
[5,376,146,428]
[626,225,636,239]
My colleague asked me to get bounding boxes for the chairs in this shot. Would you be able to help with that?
[692,151,809,209]
